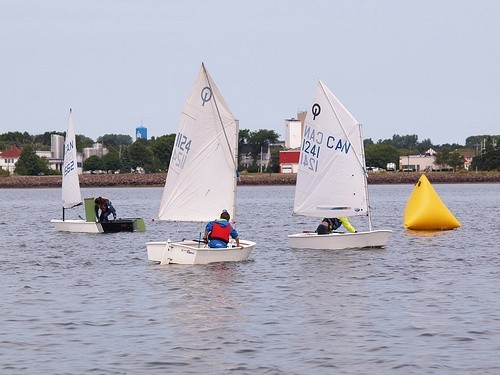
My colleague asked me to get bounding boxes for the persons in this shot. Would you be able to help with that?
[204,212,244,249]
[95,196,117,222]
[317,207,358,234]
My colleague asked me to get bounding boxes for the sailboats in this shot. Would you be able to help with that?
[288,79,393,252]
[145,63,257,265]
[50,108,146,235]
